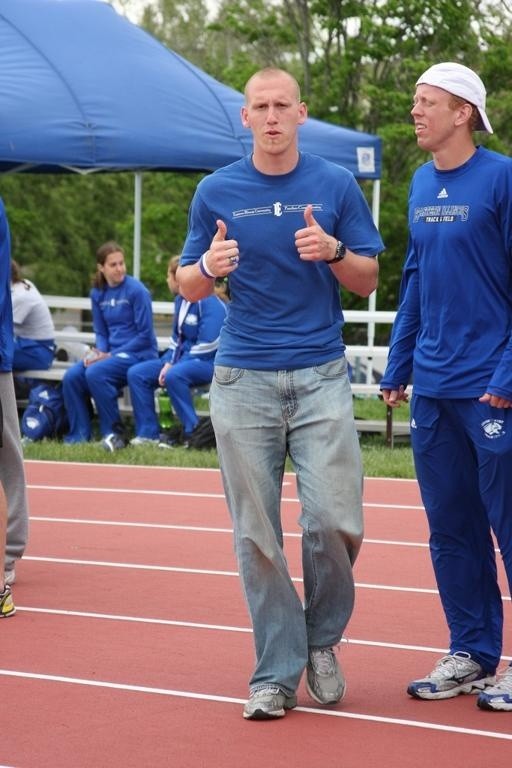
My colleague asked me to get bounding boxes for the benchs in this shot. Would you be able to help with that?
[14,294,414,451]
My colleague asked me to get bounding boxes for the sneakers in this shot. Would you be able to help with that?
[306,644,346,707]
[405,653,500,700]
[1,567,20,619]
[95,433,178,451]
[476,659,511,712]
[241,688,299,722]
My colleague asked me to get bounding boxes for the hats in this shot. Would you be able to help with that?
[414,61,496,138]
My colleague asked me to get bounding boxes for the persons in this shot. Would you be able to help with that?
[62,241,158,444]
[127,255,230,450]
[10,259,57,371]
[378,61,512,712]
[175,67,388,721]
[0,395,17,618]
[0,198,30,585]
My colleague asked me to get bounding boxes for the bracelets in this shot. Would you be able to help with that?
[199,250,217,279]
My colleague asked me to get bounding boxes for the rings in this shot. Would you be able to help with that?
[229,256,237,266]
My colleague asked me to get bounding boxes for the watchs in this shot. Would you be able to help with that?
[325,241,346,265]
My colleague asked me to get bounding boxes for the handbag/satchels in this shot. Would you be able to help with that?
[190,416,219,450]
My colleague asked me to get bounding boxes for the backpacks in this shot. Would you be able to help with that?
[21,384,67,441]
